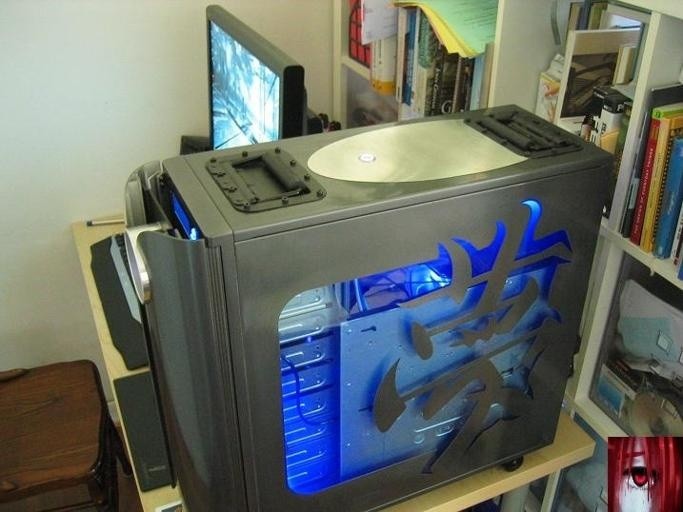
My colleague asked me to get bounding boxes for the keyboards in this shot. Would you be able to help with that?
[110,232,141,325]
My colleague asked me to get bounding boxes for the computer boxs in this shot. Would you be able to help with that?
[121,102,617,512]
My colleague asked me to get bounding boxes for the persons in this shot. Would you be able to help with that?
[606,437,683,512]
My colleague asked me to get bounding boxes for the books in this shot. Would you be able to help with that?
[368,0,494,123]
[537,25,647,219]
[621,84,683,283]
[601,357,638,402]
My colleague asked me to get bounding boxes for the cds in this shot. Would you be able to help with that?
[629,392,682,438]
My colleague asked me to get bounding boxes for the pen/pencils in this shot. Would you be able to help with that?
[86,220,125,224]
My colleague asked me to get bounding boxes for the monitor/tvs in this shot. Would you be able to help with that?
[205,4,324,150]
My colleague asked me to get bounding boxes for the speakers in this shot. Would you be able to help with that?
[125,159,164,226]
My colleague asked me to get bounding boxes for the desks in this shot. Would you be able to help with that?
[70,213,596,511]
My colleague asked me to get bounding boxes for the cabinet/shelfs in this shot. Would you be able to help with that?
[329,0,682,512]
